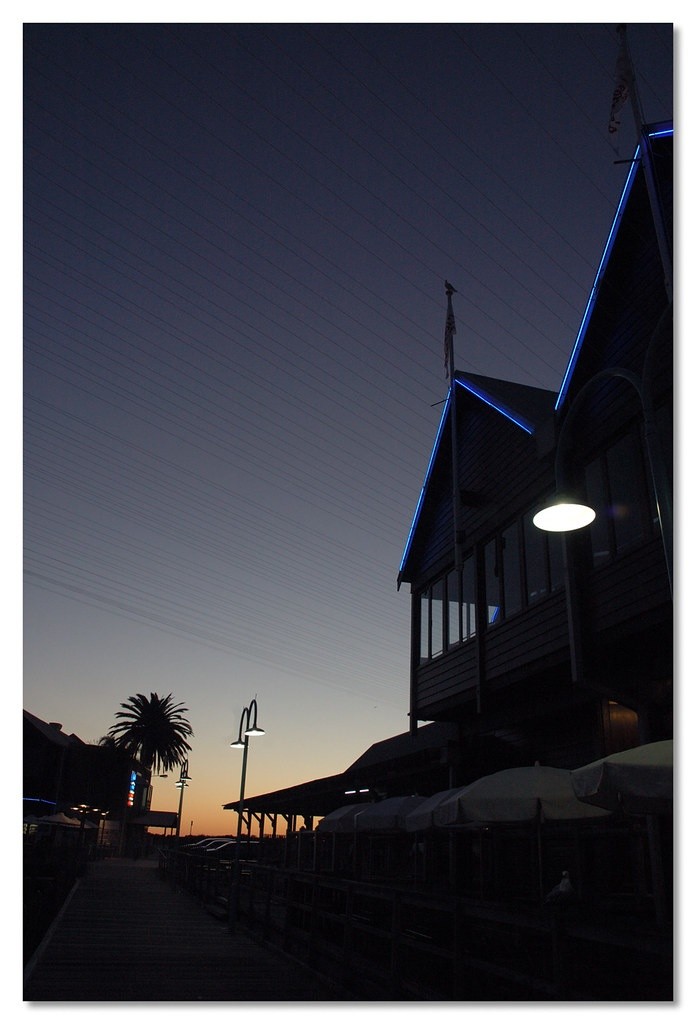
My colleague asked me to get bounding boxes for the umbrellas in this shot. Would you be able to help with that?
[319,783,483,890]
[24,812,100,828]
[434,761,612,912]
[570,738,672,816]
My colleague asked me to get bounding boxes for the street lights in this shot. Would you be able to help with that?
[533,303,673,601]
[173,759,191,861]
[224,699,265,932]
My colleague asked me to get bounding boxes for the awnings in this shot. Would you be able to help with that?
[126,811,177,828]
[224,774,384,816]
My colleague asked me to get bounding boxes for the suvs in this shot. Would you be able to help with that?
[184,838,260,862]
